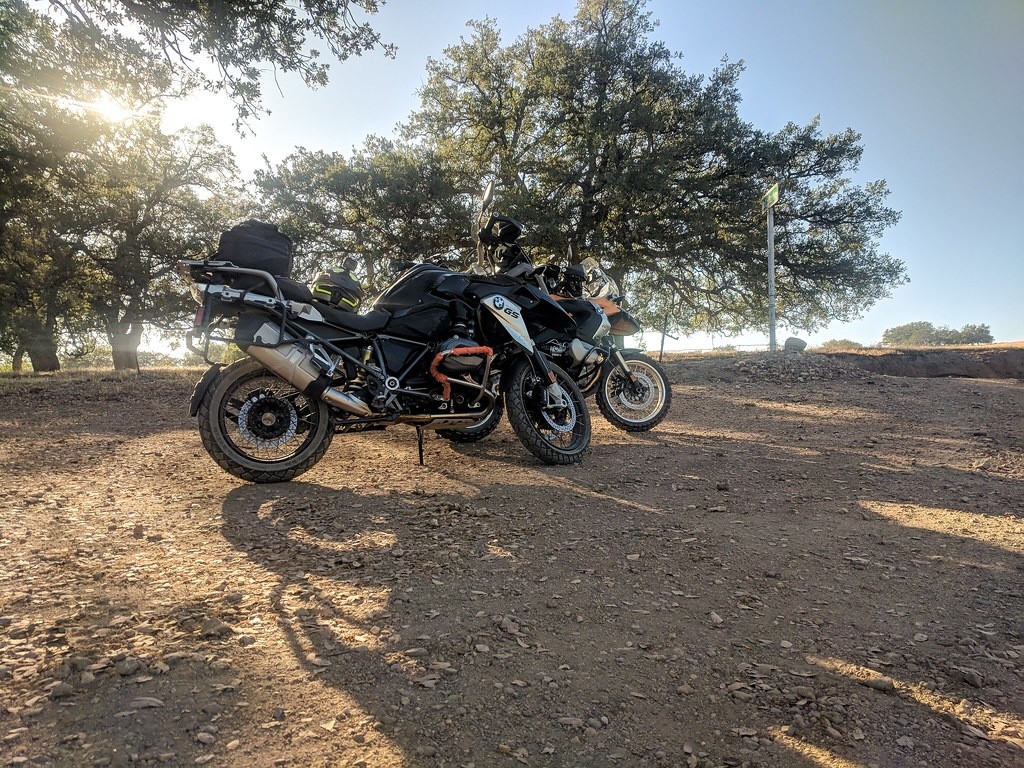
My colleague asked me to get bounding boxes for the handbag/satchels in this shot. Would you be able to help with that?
[214,220,293,278]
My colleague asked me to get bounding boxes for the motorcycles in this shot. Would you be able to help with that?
[175,181,599,479]
[423,248,670,441]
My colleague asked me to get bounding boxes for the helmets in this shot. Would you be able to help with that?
[314,267,363,313]
